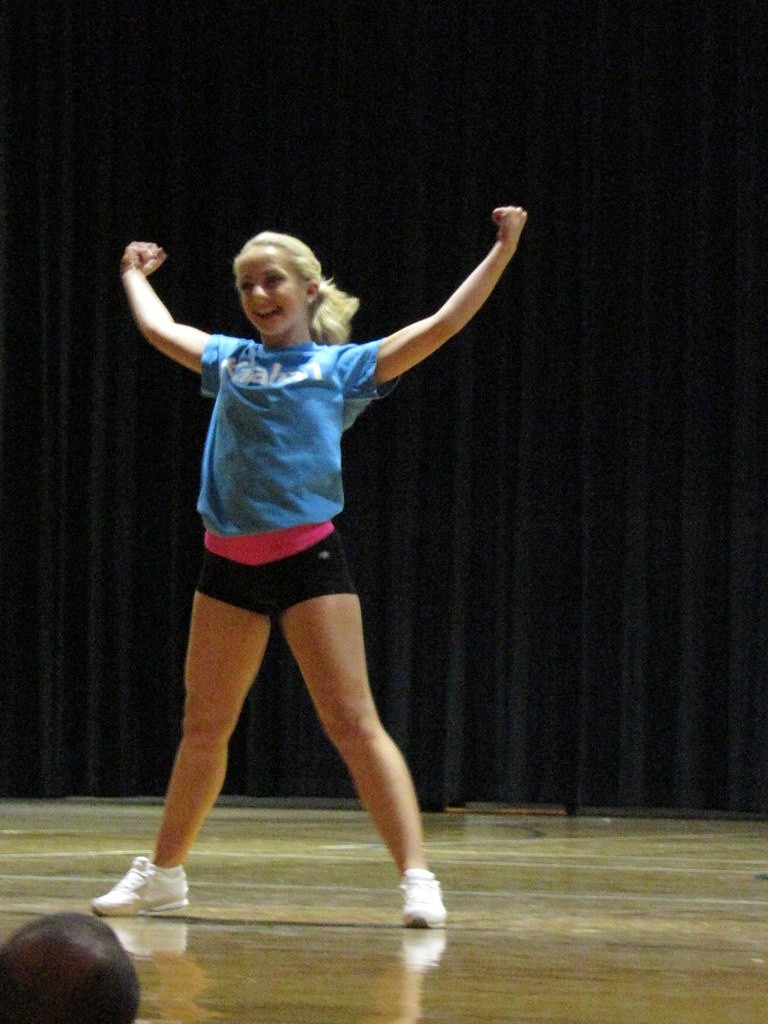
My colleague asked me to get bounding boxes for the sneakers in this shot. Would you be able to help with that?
[397,881,448,928]
[403,931,447,969]
[99,919,188,958]
[90,856,190,916]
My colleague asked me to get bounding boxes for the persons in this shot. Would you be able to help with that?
[91,205,527,929]
[0,911,138,1024]
